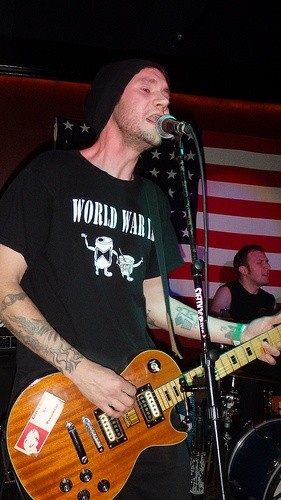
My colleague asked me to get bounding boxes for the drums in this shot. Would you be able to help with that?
[262,390,281,418]
[222,417,281,500]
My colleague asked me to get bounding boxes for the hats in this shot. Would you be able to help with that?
[89,59,167,133]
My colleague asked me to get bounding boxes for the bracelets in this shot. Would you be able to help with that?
[231,323,246,346]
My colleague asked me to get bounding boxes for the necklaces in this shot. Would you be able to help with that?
[243,284,258,294]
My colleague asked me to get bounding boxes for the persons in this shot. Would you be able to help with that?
[208,245,281,426]
[0,58,281,500]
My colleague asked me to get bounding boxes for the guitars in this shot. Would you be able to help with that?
[1,324,281,500]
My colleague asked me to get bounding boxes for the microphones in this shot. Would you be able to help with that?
[156,114,193,139]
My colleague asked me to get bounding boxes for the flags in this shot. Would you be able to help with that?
[53,115,281,368]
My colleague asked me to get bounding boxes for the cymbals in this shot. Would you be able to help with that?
[213,313,251,323]
[227,369,280,384]
